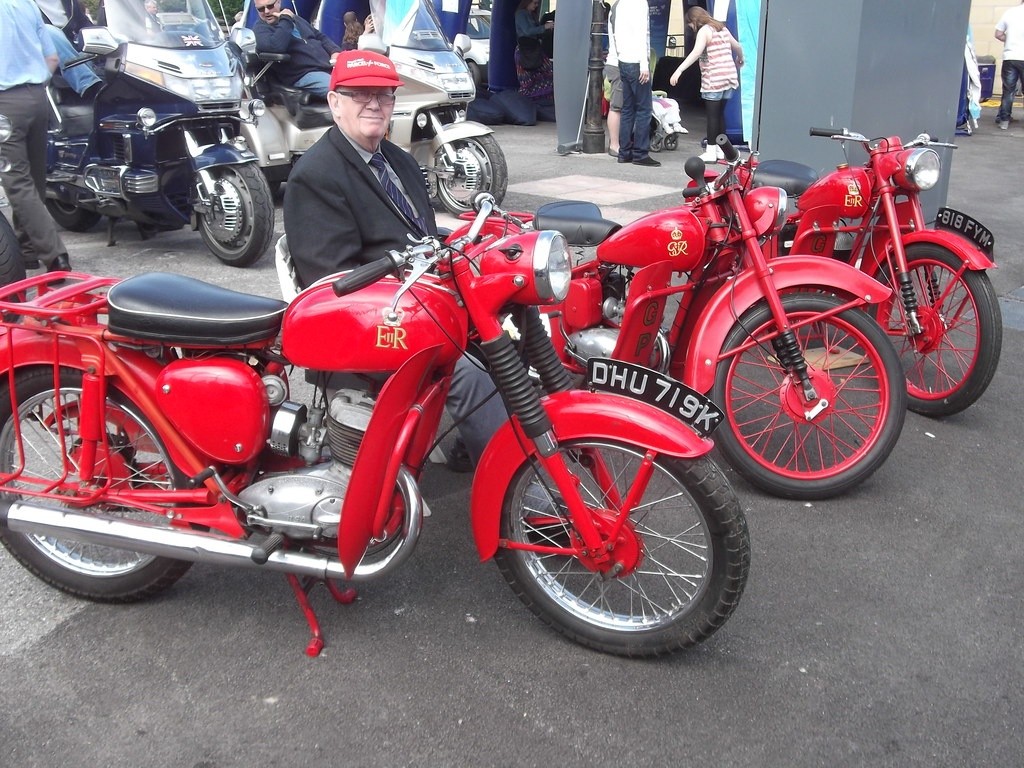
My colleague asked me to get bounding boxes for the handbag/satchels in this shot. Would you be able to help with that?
[517,36,543,71]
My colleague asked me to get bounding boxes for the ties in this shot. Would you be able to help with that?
[371,153,428,240]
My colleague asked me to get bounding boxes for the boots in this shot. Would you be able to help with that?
[699,144,725,163]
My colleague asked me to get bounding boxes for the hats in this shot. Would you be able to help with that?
[362,7,371,24]
[330,50,405,93]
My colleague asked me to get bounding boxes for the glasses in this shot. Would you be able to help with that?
[256,0,277,12]
[336,90,395,105]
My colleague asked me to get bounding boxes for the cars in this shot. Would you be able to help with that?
[460,8,491,84]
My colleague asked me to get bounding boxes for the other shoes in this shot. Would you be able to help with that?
[662,123,675,134]
[998,119,1010,130]
[618,155,633,163]
[448,437,477,473]
[632,156,661,167]
[673,123,689,134]
[25,259,40,269]
[46,253,72,285]
[995,116,1013,123]
[524,496,583,544]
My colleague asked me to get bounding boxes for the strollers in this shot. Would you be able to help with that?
[651,91,679,152]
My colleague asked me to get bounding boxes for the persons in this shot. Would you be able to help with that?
[995,0,1024,130]
[514,0,554,99]
[0,0,72,287]
[231,12,245,33]
[253,0,342,99]
[143,0,161,36]
[602,0,661,167]
[283,51,576,547]
[669,6,744,163]
[341,12,378,54]
[34,0,105,97]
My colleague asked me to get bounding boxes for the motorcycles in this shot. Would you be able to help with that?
[226,0,508,216]
[0,192,751,658]
[45,0,275,267]
[750,126,1002,419]
[444,136,910,498]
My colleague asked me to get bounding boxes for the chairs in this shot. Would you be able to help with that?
[274,232,376,391]
